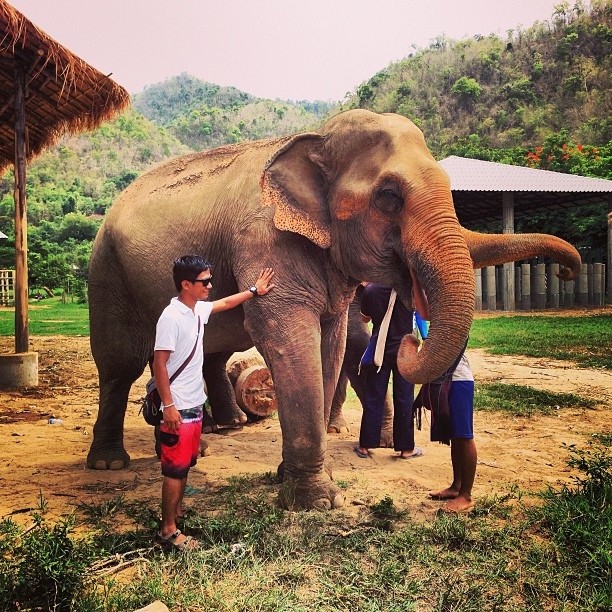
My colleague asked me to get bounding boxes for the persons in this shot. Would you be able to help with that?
[150,253,277,550]
[410,268,477,514]
[353,281,426,459]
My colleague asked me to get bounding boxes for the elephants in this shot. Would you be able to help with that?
[85,107,480,515]
[327,194,583,451]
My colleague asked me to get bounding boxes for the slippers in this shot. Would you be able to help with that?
[401,447,422,458]
[353,445,369,458]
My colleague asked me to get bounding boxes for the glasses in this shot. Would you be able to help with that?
[189,275,213,287]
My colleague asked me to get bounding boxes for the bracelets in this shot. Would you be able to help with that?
[163,403,176,408]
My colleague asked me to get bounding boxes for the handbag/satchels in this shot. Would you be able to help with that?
[138,375,162,426]
[410,372,452,446]
[357,333,388,374]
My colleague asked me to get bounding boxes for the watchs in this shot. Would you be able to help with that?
[249,285,259,297]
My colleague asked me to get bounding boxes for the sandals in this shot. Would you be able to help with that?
[152,528,194,552]
